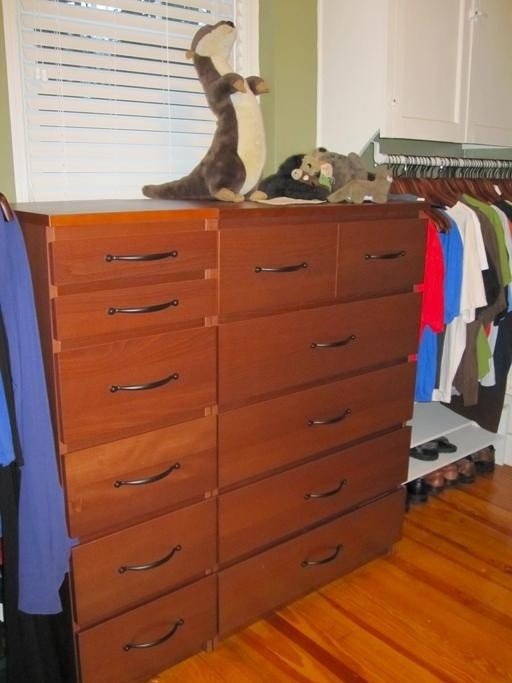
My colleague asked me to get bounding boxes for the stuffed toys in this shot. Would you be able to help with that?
[140,18,271,205]
[257,153,329,201]
[291,147,368,190]
[326,166,396,204]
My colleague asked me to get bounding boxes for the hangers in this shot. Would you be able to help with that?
[387,151,512,231]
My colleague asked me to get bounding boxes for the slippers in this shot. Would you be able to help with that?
[430,436,457,453]
[408,441,439,461]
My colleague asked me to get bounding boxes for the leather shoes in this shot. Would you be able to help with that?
[406,445,495,501]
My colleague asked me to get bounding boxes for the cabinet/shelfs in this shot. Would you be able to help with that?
[9,195,429,682]
[323,0,512,146]
